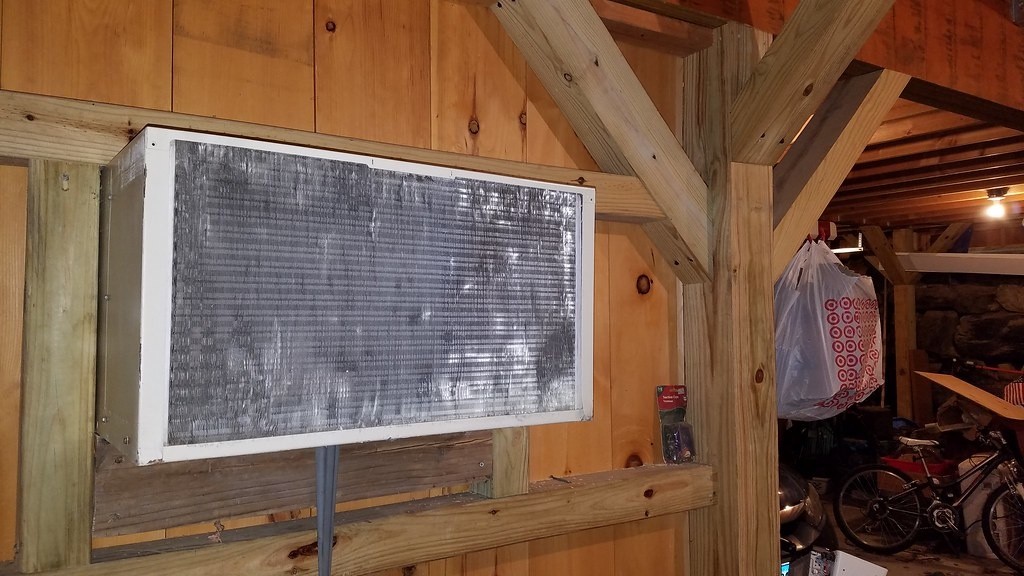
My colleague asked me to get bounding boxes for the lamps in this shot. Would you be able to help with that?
[985,187,1010,219]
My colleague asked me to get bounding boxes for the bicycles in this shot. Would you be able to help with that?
[833,436,1024,575]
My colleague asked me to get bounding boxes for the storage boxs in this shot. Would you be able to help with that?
[877,453,961,499]
